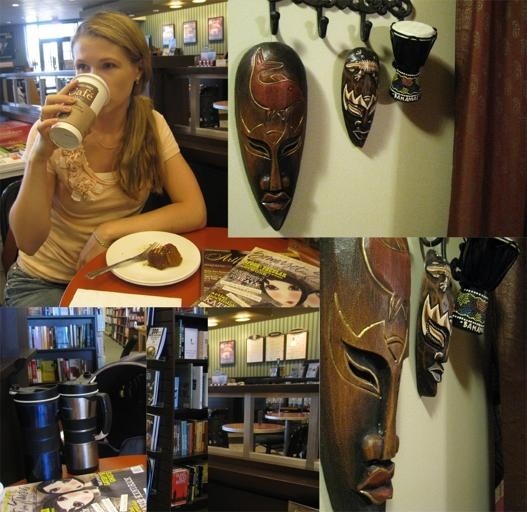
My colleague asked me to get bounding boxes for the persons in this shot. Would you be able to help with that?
[37,470,147,512]
[235,42,307,231]
[320,238,411,512]
[3,10,208,307]
[341,48,380,148]
[416,256,454,397]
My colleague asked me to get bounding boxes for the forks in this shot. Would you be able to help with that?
[85,241,162,281]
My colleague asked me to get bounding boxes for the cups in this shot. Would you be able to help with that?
[8,381,63,484]
[56,370,113,477]
[47,73,112,151]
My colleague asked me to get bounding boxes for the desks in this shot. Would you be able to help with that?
[223,407,309,455]
[2,455,147,512]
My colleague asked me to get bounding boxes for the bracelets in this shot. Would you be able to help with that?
[92,231,112,248]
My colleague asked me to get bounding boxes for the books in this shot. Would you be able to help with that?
[28,358,86,384]
[29,323,95,350]
[189,246,320,308]
[27,308,94,318]
[146,327,208,360]
[0,464,147,512]
[146,413,208,456]
[147,458,208,507]
[106,307,144,349]
[146,363,208,410]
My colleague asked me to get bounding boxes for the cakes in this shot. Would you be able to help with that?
[147,244,182,269]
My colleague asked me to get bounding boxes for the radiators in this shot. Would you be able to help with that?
[61,224,319,311]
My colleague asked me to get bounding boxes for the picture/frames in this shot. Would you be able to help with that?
[219,340,237,366]
[208,15,224,42]
[183,19,198,45]
[162,23,175,47]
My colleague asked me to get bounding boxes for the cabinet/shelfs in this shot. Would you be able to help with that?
[154,64,229,155]
[107,309,146,350]
[27,314,98,376]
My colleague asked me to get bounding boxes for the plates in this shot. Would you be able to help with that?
[105,229,202,288]
